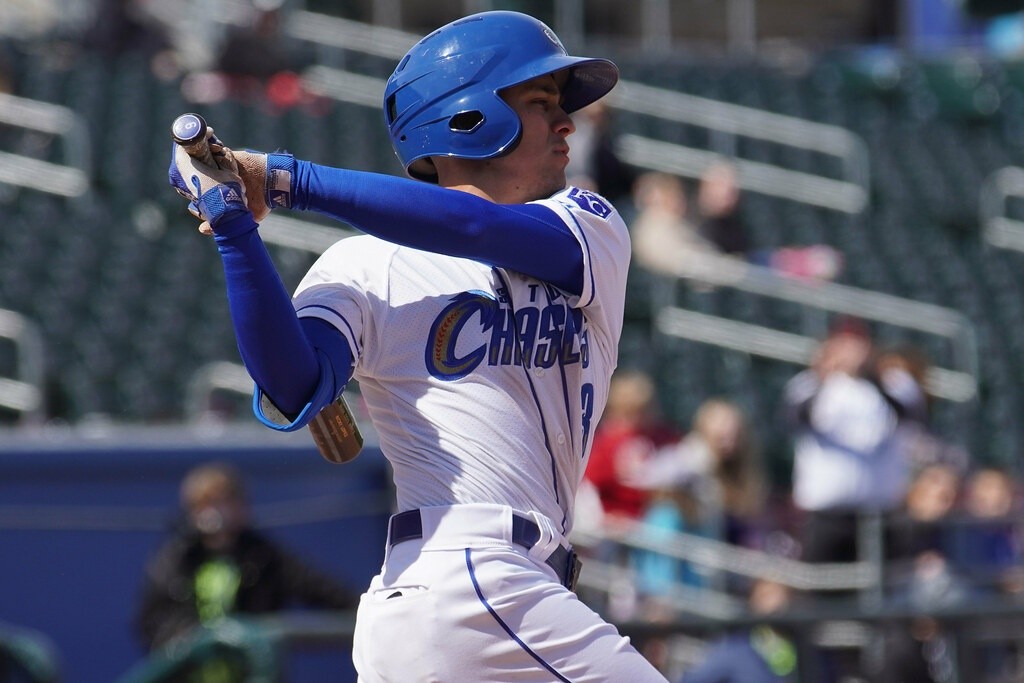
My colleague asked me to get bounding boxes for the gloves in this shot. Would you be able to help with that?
[169,126,249,227]
[188,149,295,236]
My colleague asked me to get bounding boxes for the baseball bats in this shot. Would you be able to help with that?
[170,113,366,468]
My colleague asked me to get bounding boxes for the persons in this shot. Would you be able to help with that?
[0,0,1024,683]
[160,9,683,682]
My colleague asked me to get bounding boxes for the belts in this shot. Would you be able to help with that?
[390,509,583,593]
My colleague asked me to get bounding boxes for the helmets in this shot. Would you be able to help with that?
[383,10,619,183]
